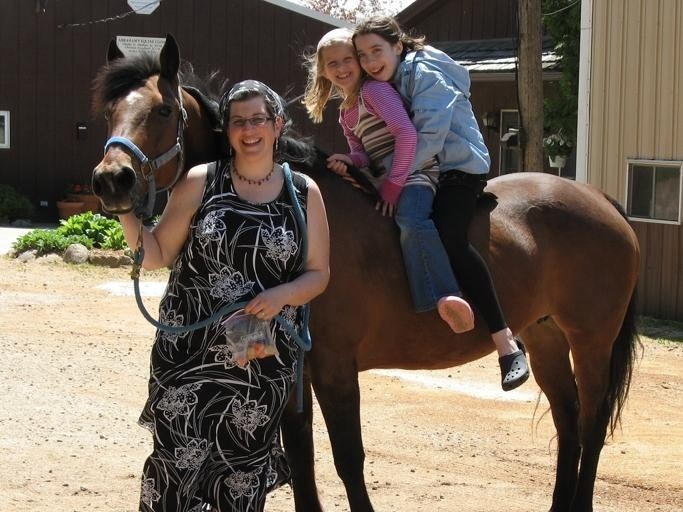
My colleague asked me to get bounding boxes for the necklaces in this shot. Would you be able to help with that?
[231,157,274,185]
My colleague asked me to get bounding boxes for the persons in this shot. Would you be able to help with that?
[112,79,331,512]
[297,25,476,333]
[353,12,530,390]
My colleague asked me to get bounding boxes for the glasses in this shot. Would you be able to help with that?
[227,115,270,128]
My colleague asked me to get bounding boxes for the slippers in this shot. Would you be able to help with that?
[437,294,475,334]
[497,338,529,392]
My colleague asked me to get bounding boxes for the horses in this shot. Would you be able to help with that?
[90,31,644,511]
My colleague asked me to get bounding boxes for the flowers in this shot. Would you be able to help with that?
[66,184,91,195]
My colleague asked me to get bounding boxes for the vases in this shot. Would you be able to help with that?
[55,195,100,219]
